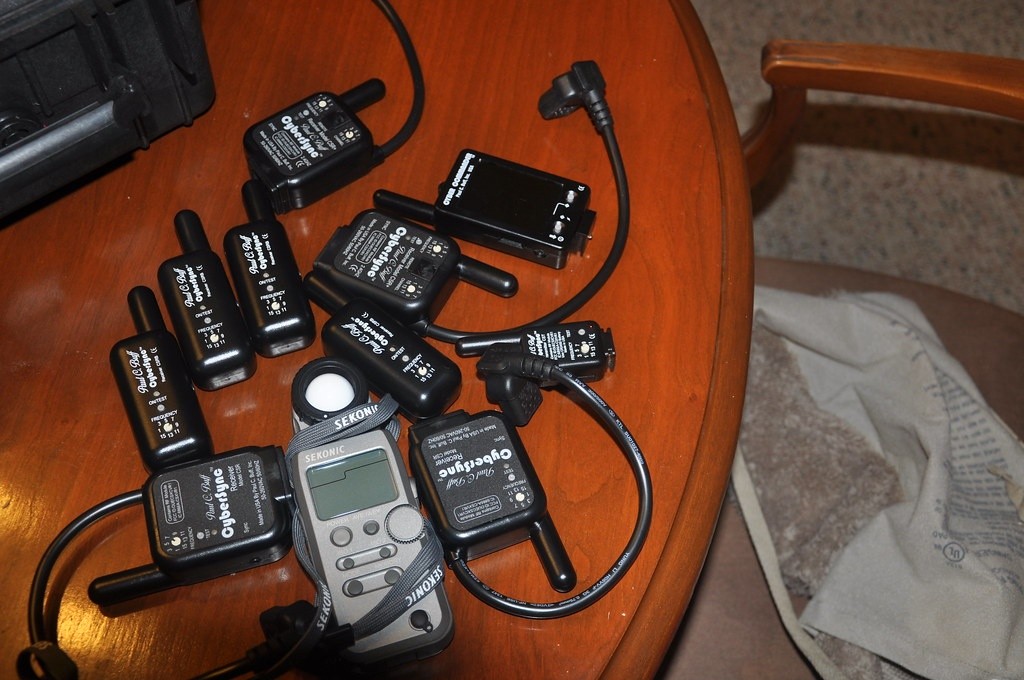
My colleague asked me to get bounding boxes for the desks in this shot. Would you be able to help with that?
[1,0,755,679]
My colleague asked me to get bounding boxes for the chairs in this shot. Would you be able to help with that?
[656,41,1023,680]
